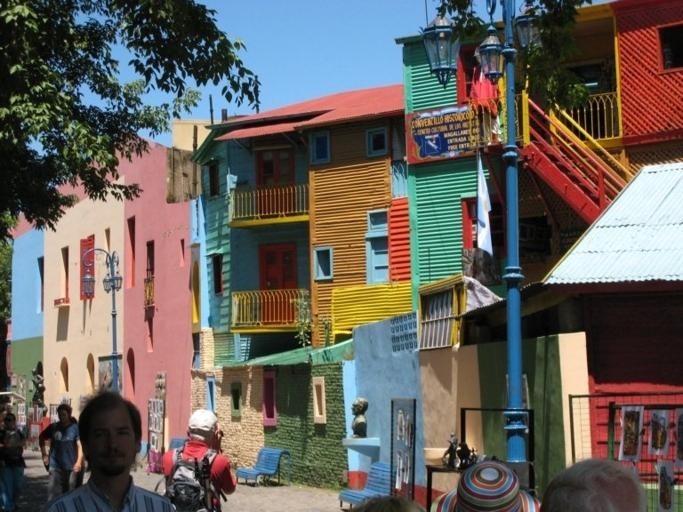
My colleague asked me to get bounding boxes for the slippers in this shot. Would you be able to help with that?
[164,448,220,512]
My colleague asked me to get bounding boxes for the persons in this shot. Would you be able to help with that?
[0,395,239,511]
[350,398,368,436]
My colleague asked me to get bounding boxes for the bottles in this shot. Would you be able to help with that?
[189,409,217,431]
[430,461,541,512]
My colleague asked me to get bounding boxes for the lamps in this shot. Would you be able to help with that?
[338,462,398,509]
[235,447,290,486]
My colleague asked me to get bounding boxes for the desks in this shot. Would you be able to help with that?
[421,0,531,486]
[79,245,123,398]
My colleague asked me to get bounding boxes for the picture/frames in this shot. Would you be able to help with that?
[425,464,462,512]
